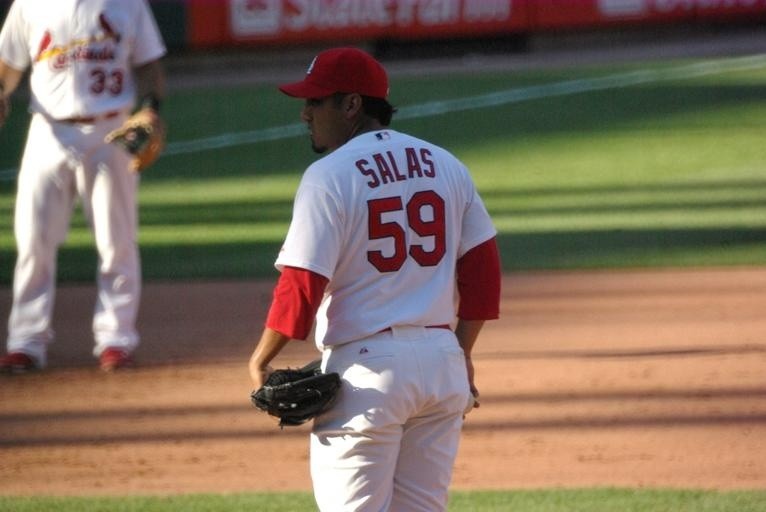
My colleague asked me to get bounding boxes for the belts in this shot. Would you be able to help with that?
[381,325,450,334]
[70,113,119,123]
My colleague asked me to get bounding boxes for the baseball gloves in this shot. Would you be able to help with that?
[250,359,340,425]
[104,106,163,173]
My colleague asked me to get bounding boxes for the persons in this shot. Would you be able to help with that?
[247,46,505,512]
[0,2,169,370]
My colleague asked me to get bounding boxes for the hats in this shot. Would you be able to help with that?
[278,47,390,101]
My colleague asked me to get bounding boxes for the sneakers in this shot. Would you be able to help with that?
[97,350,133,375]
[2,348,43,379]
